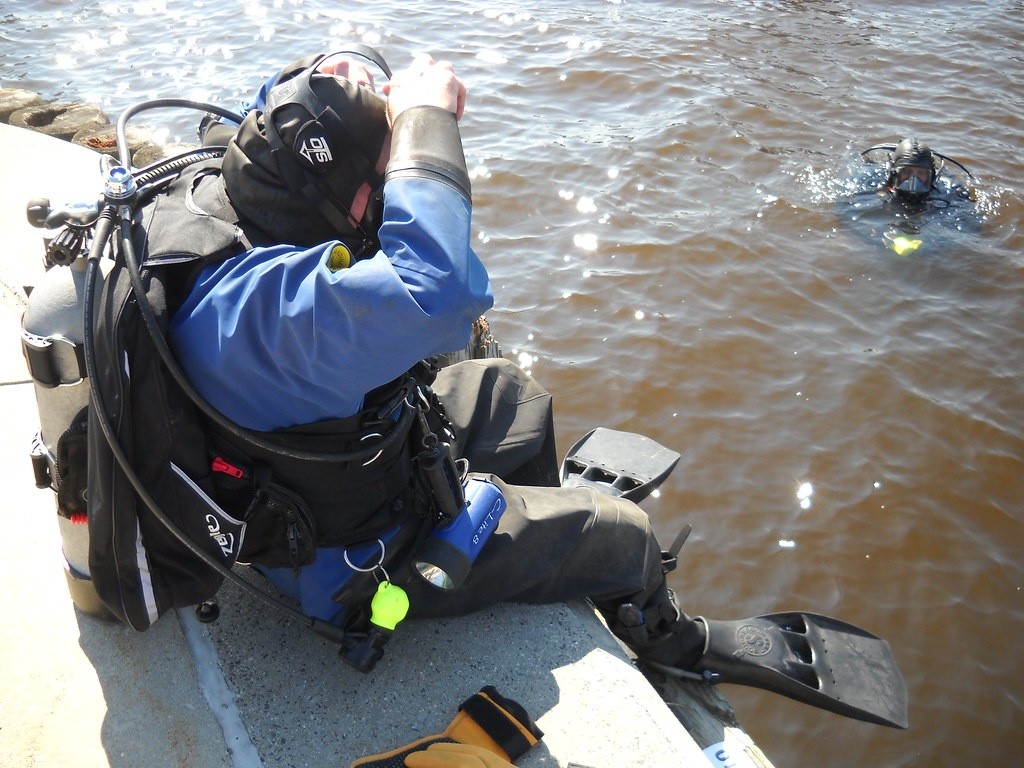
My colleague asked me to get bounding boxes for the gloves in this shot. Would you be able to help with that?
[350,685,544,767]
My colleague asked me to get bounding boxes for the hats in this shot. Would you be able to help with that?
[892,138,933,168]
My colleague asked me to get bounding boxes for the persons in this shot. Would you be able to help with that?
[841,135,983,257]
[144,55,781,732]
